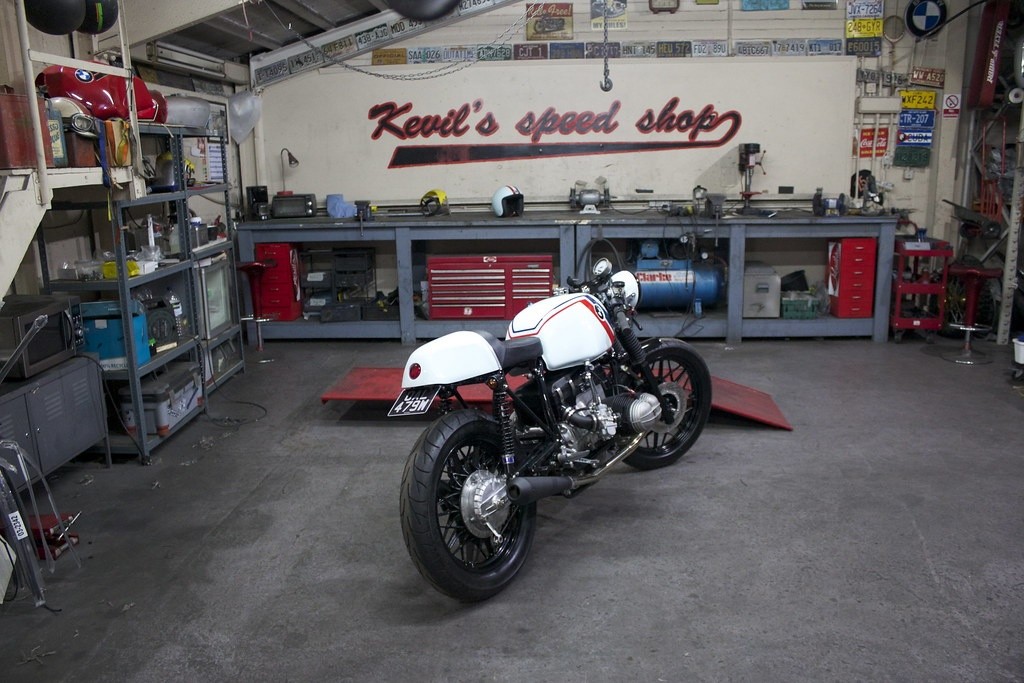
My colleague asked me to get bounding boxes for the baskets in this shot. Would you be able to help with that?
[781,292,819,319]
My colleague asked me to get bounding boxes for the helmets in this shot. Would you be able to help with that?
[420,190,447,216]
[492,185,524,216]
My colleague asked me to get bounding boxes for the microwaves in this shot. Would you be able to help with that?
[272,193,317,219]
[0,294,85,380]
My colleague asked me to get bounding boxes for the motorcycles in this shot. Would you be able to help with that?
[384,255,714,601]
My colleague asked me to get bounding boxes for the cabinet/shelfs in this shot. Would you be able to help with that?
[0,115,955,498]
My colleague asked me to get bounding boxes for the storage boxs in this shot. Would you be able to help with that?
[743,260,783,317]
[78,300,152,370]
[361,297,401,321]
[335,272,373,286]
[1,93,57,169]
[338,255,371,271]
[321,302,363,322]
[782,300,818,319]
[303,292,332,313]
[299,270,332,288]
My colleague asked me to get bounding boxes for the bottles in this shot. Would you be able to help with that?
[164,287,185,337]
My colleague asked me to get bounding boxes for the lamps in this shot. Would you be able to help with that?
[276,148,299,195]
